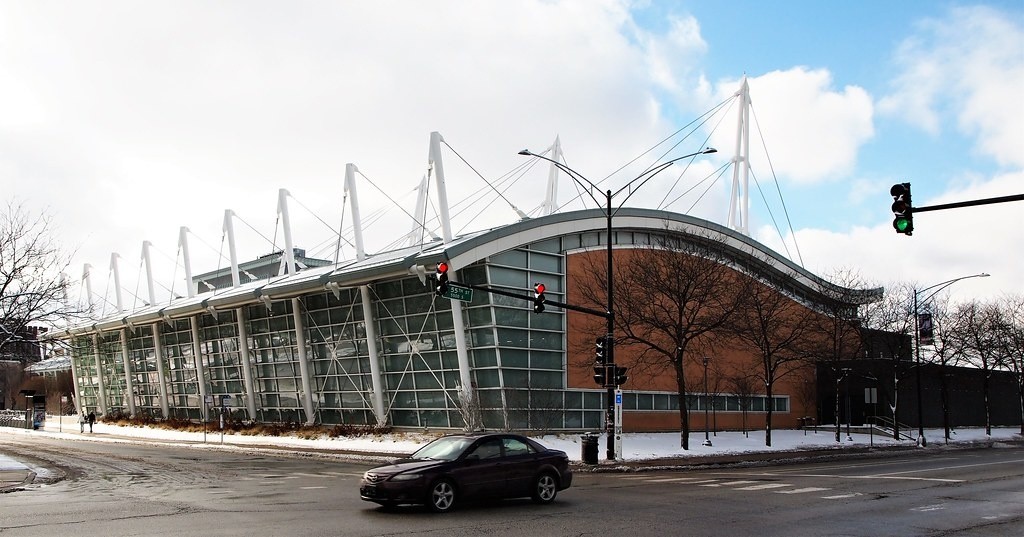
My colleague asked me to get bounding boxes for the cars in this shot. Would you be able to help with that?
[360,430,573,514]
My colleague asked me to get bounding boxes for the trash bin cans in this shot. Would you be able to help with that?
[581,436,599,465]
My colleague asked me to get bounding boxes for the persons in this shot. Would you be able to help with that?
[77,410,95,434]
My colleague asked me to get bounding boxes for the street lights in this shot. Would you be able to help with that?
[913,272,991,448]
[841,368,853,441]
[699,357,719,443]
[518,147,717,461]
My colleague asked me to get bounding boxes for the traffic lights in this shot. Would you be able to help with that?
[534,281,547,314]
[595,336,606,365]
[593,365,607,385]
[435,259,449,296]
[613,365,629,387]
[890,181,914,237]
